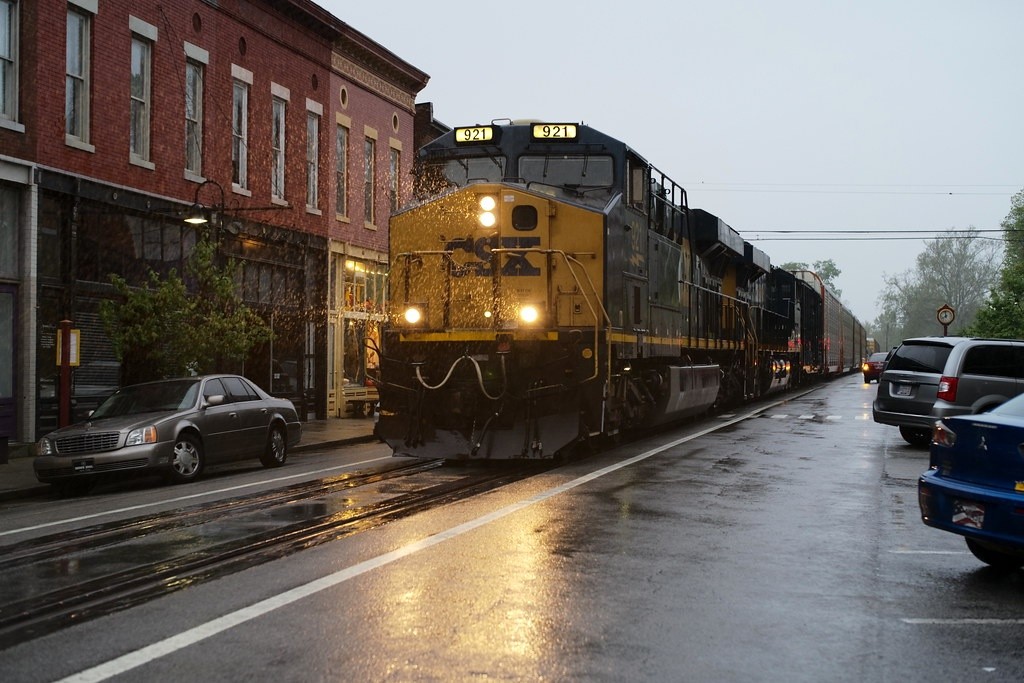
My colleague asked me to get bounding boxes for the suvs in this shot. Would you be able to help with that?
[872,333,1024,445]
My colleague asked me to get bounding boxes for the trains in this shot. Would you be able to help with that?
[361,116,881,465]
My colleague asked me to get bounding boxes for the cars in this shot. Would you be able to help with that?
[863,352,889,383]
[883,346,899,371]
[916,391,1024,568]
[33,373,303,496]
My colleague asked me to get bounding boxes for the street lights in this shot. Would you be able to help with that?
[183,178,226,264]
